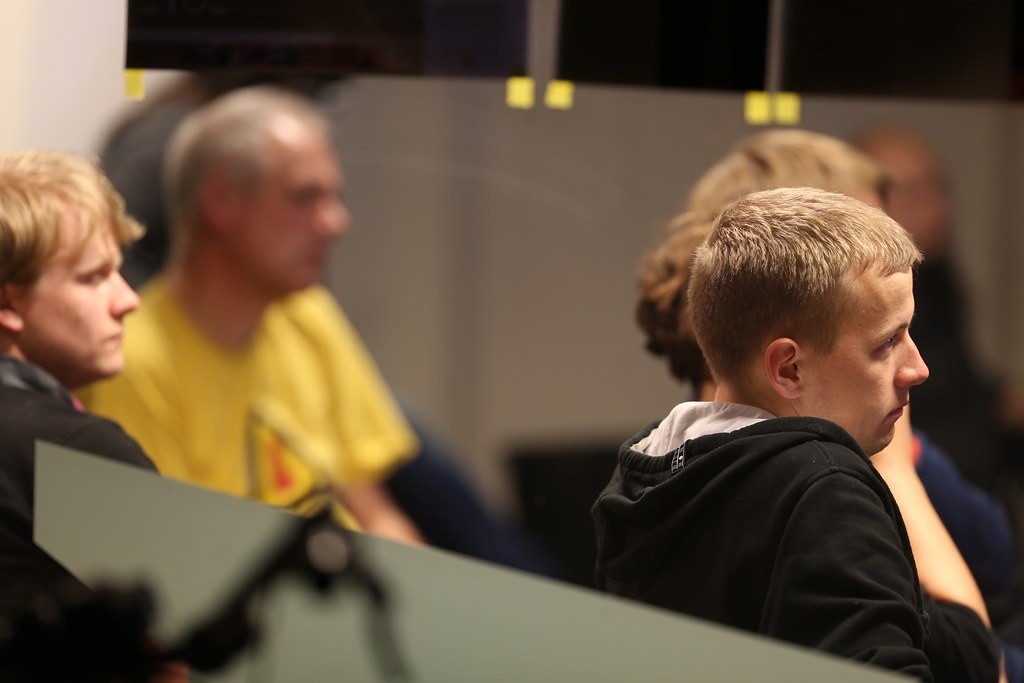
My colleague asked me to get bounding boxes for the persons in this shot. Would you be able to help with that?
[0,151,161,682]
[591,127,1023,682]
[94,71,553,581]
